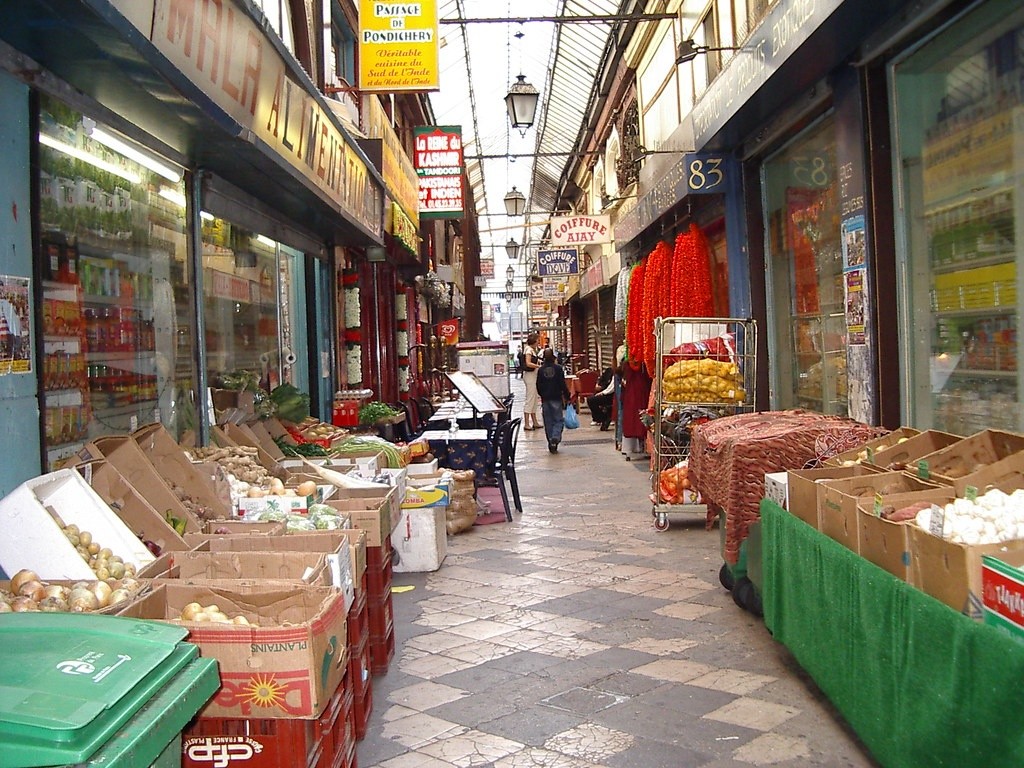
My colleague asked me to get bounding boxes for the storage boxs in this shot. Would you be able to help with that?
[765,424,1024,639]
[0,389,447,768]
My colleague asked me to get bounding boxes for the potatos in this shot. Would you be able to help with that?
[663,358,744,402]
[163,477,219,527]
[172,602,260,628]
[294,416,338,440]
[54,517,136,580]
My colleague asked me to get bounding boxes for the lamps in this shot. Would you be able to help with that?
[674,39,740,66]
[628,145,696,163]
[503,73,540,138]
[601,194,637,210]
[504,185,527,217]
[505,238,520,259]
[38,115,277,250]
[504,265,515,303]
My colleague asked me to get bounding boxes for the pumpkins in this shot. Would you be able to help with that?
[246,478,317,501]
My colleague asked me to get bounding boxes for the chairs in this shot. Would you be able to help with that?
[422,397,435,419]
[397,400,424,438]
[386,403,419,443]
[474,393,524,523]
[576,368,600,414]
[409,397,430,431]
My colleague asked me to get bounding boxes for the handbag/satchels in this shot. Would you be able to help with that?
[564,402,579,429]
[521,353,538,372]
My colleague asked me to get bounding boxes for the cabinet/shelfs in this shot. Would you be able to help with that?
[790,313,848,418]
[75,229,229,439]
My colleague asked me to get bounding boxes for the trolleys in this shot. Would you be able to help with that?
[651,315,757,532]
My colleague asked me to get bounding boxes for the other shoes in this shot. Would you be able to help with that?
[524,426,535,430]
[532,425,543,428]
[549,439,558,454]
[590,420,600,426]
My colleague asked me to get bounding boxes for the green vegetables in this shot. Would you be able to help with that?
[214,370,278,416]
[357,402,401,424]
[272,433,336,457]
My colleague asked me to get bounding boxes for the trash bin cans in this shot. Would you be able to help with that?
[1,609,224,768]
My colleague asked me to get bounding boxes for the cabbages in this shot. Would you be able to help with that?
[258,504,340,532]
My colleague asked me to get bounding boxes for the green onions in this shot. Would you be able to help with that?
[267,382,312,424]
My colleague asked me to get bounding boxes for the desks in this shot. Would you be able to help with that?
[564,374,602,412]
[428,401,495,466]
[759,498,1024,768]
[418,429,495,488]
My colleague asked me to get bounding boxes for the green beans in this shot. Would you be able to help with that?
[331,442,405,468]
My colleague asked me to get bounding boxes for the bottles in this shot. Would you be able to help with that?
[83,259,190,411]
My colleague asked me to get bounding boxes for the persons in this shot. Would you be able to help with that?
[522,334,544,430]
[515,337,553,380]
[537,349,571,453]
[587,353,615,432]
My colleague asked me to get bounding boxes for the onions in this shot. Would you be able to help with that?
[0,569,139,613]
[658,466,692,503]
